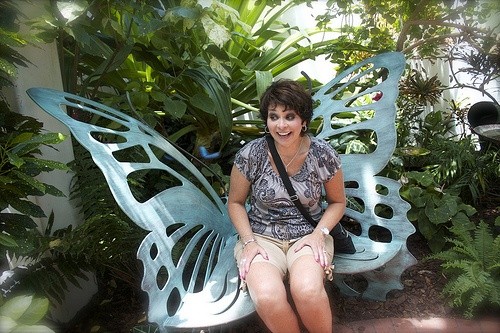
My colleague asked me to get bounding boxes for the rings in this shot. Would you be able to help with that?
[243,259,246,261]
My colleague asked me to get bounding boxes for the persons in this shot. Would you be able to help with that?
[227,78,346,333]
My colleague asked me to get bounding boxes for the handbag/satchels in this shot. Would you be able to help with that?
[329,222,356,254]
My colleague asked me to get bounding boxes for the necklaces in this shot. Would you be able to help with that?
[276,137,302,172]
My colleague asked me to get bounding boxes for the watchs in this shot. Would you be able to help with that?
[317,226,329,237]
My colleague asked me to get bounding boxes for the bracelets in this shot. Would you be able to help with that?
[242,238,254,247]
